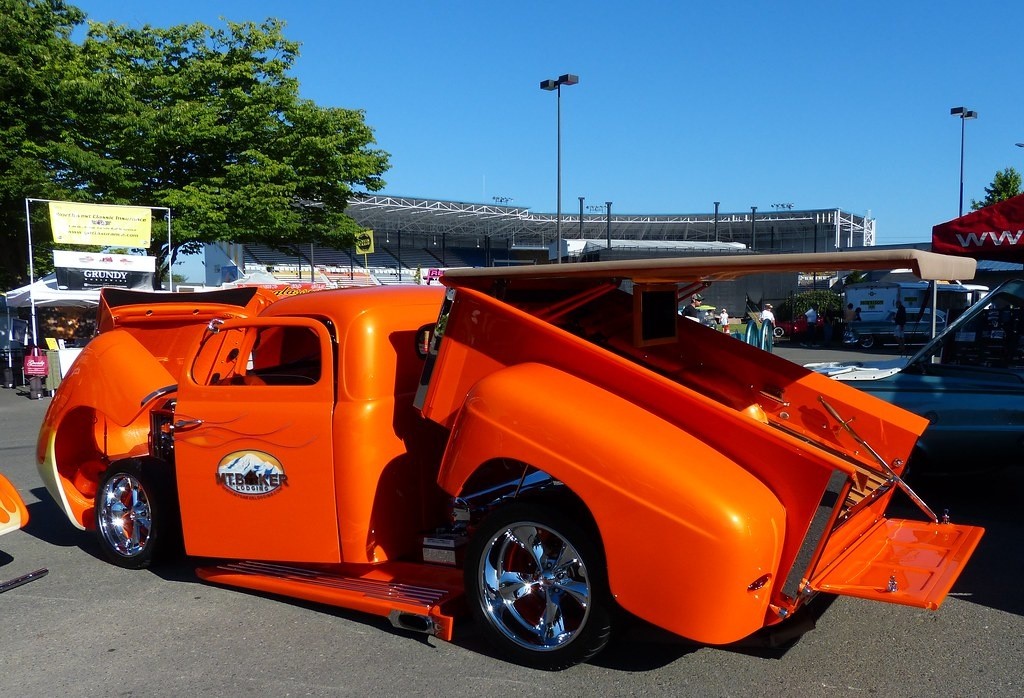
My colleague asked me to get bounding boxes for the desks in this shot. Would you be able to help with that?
[43,348,83,397]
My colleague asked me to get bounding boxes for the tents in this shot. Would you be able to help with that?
[930,191,1024,267]
[4,270,153,397]
[230,271,287,284]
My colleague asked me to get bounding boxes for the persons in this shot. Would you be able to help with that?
[892,300,905,351]
[760,303,776,345]
[682,293,705,319]
[704,310,713,316]
[845,303,861,321]
[719,308,729,333]
[799,303,820,347]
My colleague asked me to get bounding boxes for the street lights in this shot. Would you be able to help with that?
[950,106,979,217]
[539,74,580,264]
[751,206,758,252]
[577,197,586,239]
[604,201,613,249]
[712,201,721,241]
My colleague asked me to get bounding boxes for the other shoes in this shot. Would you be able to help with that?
[800,343,807,347]
[812,344,820,347]
[897,346,905,351]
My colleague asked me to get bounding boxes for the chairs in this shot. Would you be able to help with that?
[253,324,321,370]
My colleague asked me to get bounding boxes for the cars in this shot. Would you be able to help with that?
[841,307,953,352]
[794,278,1024,507]
[772,315,824,342]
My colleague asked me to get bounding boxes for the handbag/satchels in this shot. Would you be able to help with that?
[24,347,49,376]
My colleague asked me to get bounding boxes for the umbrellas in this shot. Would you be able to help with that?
[695,305,716,312]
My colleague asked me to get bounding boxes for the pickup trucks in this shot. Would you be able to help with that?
[33,248,991,674]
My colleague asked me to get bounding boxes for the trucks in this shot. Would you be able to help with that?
[844,277,992,342]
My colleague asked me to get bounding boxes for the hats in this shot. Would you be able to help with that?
[722,309,726,312]
[764,304,773,310]
[691,293,705,301]
